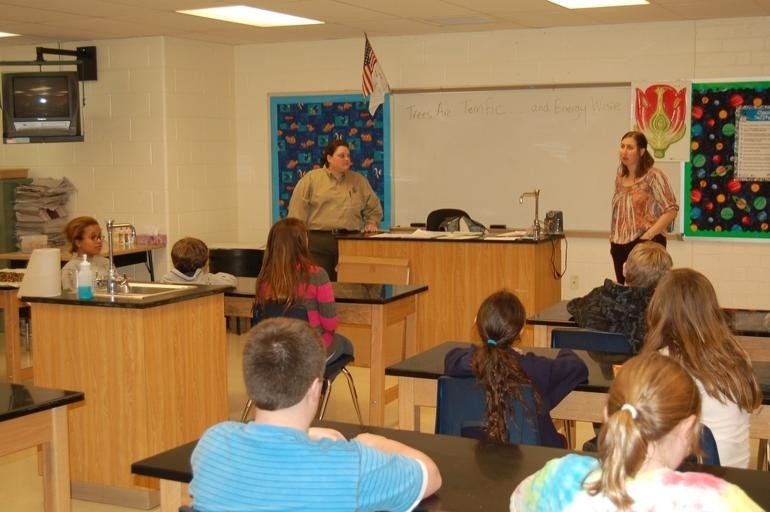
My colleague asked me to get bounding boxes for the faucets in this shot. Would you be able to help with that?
[519,189,540,226]
[106,219,136,271]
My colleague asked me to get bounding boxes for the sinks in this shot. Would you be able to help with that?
[95,282,198,302]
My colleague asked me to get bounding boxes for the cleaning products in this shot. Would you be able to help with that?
[78,254,93,300]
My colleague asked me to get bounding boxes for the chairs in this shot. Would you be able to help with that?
[426,208,488,233]
[689,422,721,468]
[434,374,542,449]
[208,249,265,336]
[240,301,363,424]
[552,329,632,356]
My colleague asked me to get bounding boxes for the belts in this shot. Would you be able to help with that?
[310,227,359,235]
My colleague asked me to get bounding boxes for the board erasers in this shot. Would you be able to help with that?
[410,223,425,227]
[490,225,506,229]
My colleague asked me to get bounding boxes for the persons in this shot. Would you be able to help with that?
[286,139,384,279]
[610,131,680,284]
[443,290,590,451]
[249,217,354,419]
[160,236,237,288]
[508,355,766,512]
[639,266,764,470]
[58,215,120,295]
[188,317,444,511]
[565,240,674,354]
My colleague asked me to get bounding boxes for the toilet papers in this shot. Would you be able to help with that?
[16,248,62,299]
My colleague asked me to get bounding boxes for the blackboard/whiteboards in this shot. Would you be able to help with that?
[390,81,632,238]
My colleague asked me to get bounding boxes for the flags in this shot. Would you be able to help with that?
[361,37,390,117]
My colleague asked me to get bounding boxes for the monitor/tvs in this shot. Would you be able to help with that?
[2,71,82,136]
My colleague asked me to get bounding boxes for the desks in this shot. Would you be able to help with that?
[18,279,236,511]
[526,299,770,349]
[223,275,429,432]
[0,267,35,386]
[0,236,166,282]
[385,341,769,473]
[131,416,769,511]
[0,380,86,511]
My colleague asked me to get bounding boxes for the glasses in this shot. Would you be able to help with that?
[80,235,105,241]
